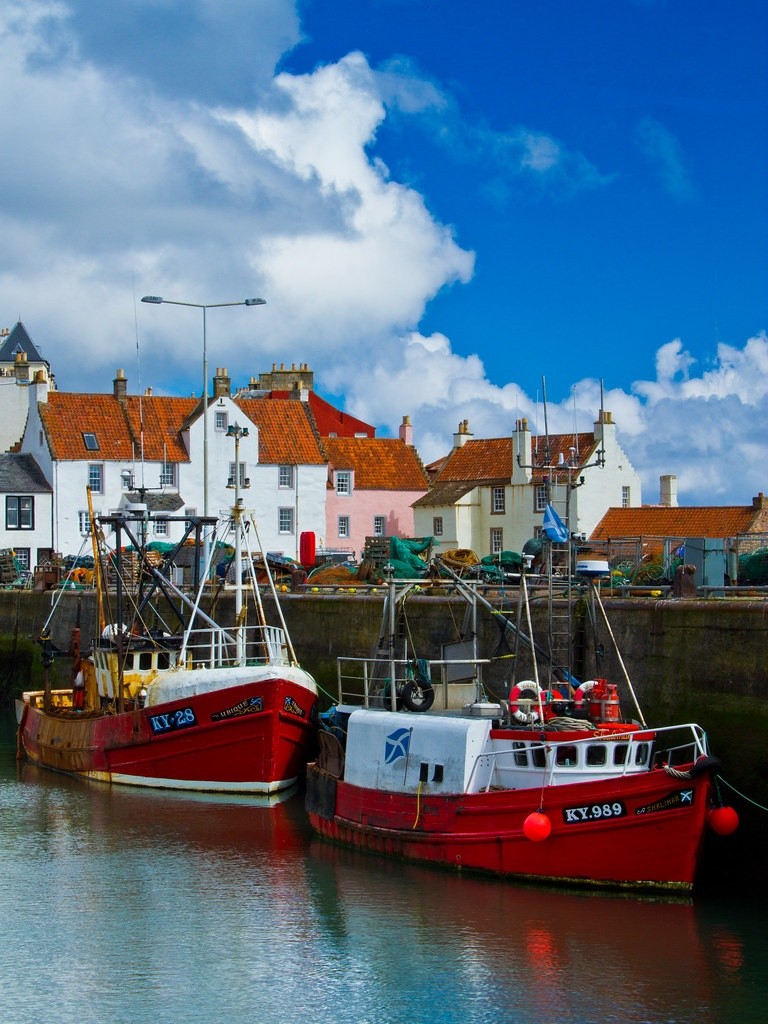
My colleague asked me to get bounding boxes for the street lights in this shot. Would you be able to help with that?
[141,294,269,582]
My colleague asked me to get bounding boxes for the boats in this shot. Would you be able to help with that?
[306,375,721,902]
[12,420,325,810]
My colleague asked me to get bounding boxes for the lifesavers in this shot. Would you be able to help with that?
[384,681,404,712]
[511,680,545,723]
[403,680,435,712]
[574,681,602,711]
